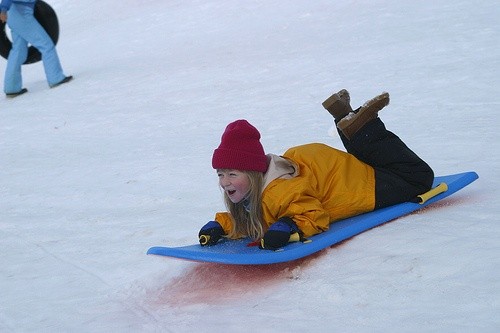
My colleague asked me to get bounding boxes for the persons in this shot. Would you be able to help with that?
[199,86,435,248]
[0,0,72,97]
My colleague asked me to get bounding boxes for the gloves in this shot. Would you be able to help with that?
[258,217,299,251]
[198,218,227,247]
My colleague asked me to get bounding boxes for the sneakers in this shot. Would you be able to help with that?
[321,87,351,119]
[334,90,391,137]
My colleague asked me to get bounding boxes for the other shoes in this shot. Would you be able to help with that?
[5,87,27,99]
[49,74,73,89]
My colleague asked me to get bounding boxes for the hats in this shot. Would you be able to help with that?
[211,119,268,173]
